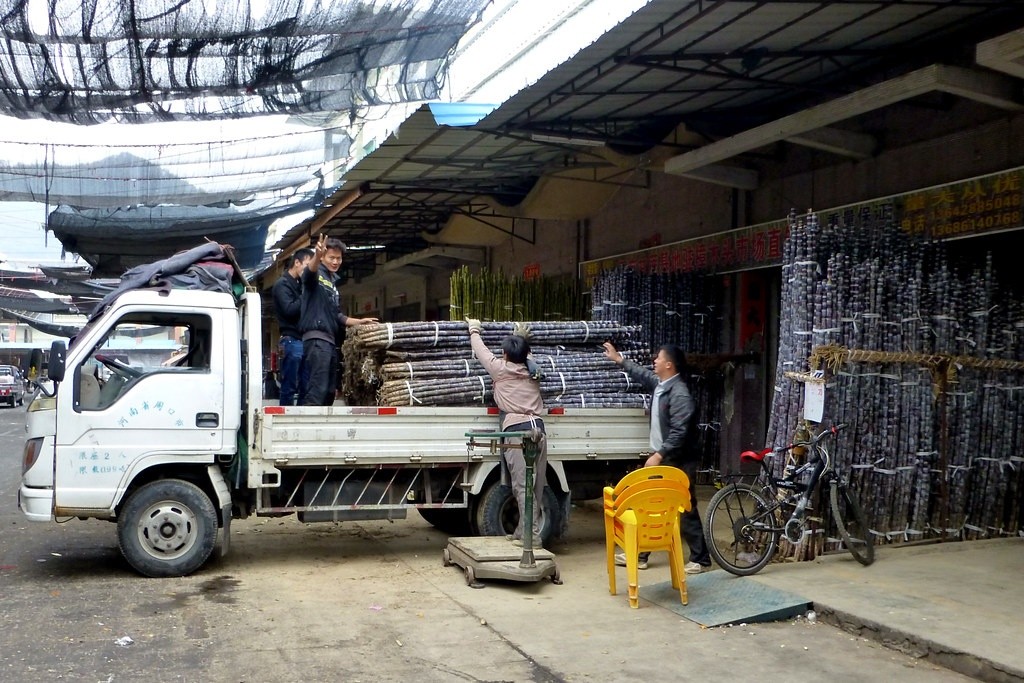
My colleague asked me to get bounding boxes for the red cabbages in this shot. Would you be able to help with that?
[742,208,1024,563]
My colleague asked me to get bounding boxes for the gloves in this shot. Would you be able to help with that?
[513,322,534,340]
[465,316,484,334]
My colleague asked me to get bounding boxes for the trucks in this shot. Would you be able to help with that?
[0,365,28,408]
[17,284,656,578]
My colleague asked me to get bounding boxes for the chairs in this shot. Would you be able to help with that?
[603,466,692,608]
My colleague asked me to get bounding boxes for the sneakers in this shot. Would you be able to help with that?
[614,552,648,569]
[684,562,713,574]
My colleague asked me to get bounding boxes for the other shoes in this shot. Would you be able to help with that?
[512,538,542,549]
[505,534,519,541]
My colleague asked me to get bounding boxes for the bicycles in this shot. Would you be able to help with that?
[695,422,875,576]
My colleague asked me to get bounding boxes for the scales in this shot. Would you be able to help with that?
[442,428,564,589]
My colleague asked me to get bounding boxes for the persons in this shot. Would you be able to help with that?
[603,342,712,573]
[466,316,547,549]
[263,372,281,399]
[273,248,315,406]
[301,233,380,405]
